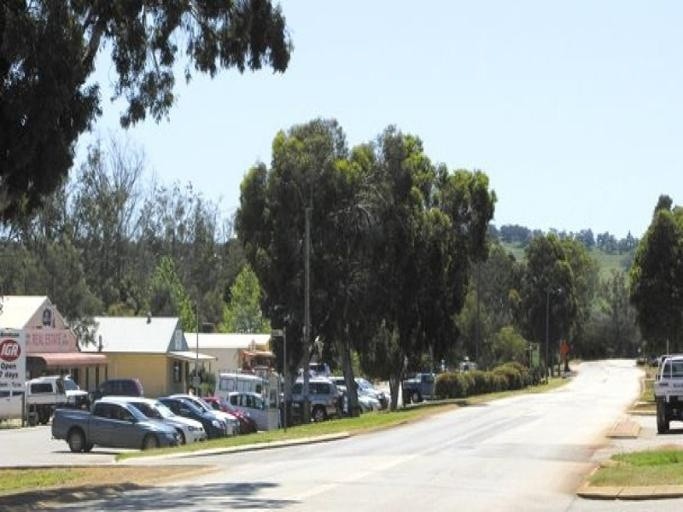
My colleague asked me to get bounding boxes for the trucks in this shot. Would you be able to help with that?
[653,350,682,434]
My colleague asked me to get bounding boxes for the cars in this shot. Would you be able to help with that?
[401,358,479,403]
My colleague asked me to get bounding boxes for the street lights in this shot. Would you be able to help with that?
[474,234,505,363]
[544,286,563,385]
[195,303,216,396]
